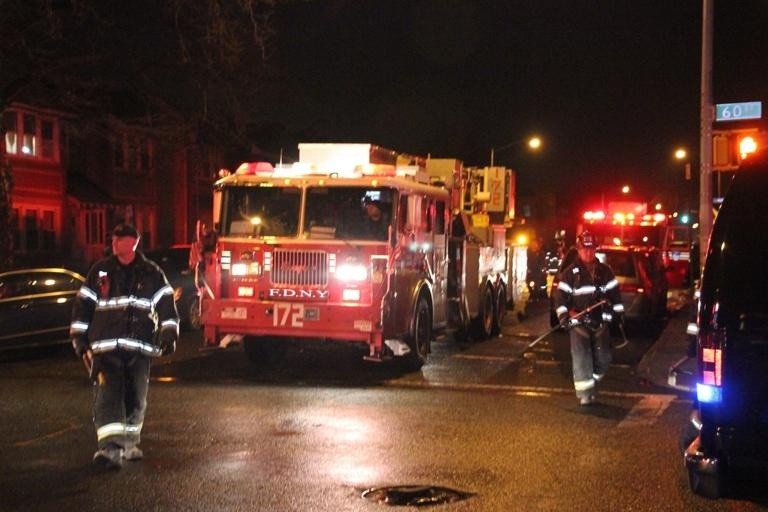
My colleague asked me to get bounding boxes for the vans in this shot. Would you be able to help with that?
[682,150,768,500]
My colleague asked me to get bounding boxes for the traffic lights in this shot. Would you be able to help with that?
[713,129,761,166]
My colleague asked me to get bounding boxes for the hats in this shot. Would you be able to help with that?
[104,223,140,239]
[576,229,599,248]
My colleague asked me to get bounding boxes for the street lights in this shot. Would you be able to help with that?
[601,184,633,214]
[490,135,540,166]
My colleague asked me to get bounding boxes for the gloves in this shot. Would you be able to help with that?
[155,331,176,356]
[613,312,625,328]
[558,312,572,329]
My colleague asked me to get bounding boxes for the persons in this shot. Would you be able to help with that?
[553,231,624,406]
[347,202,385,240]
[67,222,179,468]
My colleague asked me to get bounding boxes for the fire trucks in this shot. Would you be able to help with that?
[194,143,508,368]
[578,211,691,286]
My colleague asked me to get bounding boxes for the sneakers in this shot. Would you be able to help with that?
[93,446,123,470]
[579,395,597,406]
[124,445,144,461]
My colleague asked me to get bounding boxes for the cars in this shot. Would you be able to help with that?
[549,245,668,333]
[166,245,199,329]
[0,266,87,353]
